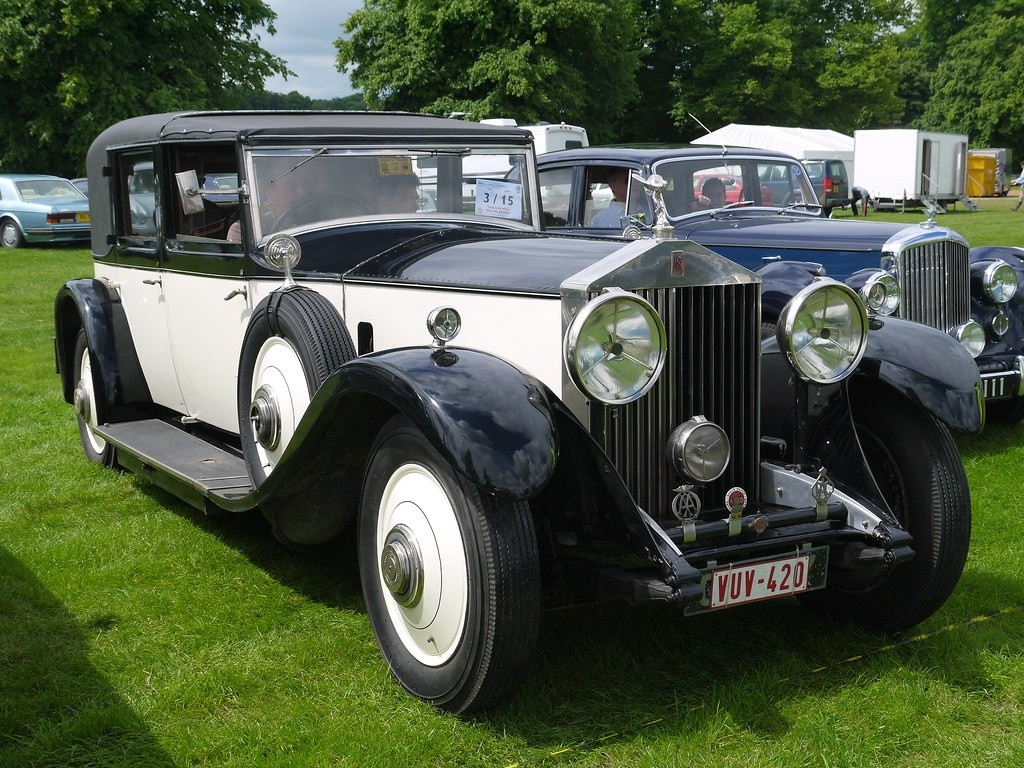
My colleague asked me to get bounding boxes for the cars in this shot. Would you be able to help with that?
[0,119,852,250]
[496,142,1024,436]
[52,105,991,723]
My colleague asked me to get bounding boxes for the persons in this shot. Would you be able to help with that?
[591,167,712,228]
[852,186,869,216]
[373,171,420,215]
[702,178,728,209]
[1010,161,1024,211]
[226,161,317,243]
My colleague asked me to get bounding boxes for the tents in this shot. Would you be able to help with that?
[690,123,854,209]
